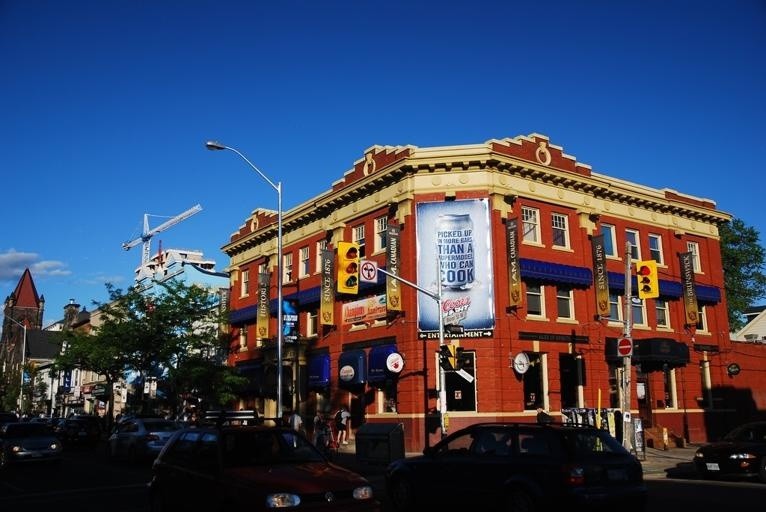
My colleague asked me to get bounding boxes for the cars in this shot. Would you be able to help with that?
[384,422,648,512]
[0,409,220,475]
[693,421,766,484]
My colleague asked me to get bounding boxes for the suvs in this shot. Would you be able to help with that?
[145,409,383,512]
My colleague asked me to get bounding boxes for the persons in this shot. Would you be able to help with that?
[535,403,555,423]
[290,405,351,445]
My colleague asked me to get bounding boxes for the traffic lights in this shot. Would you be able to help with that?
[636,260,659,299]
[336,238,357,296]
[427,388,439,400]
[439,344,454,370]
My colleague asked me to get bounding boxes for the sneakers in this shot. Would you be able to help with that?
[342,441,348,445]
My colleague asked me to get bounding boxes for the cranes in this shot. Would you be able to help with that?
[121,203,203,280]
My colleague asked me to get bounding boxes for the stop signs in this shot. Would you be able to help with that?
[615,338,632,356]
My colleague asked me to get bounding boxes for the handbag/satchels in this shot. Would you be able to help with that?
[329,440,338,448]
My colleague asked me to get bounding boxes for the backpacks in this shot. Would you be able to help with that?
[335,410,344,423]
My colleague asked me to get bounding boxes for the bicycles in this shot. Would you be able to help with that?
[313,428,339,466]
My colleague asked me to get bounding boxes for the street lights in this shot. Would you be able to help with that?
[206,142,282,433]
